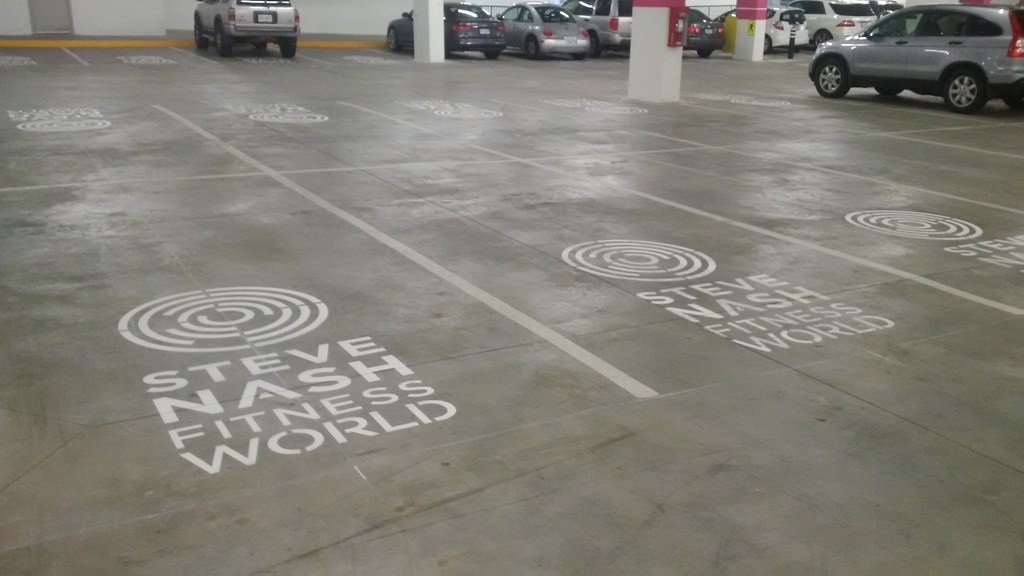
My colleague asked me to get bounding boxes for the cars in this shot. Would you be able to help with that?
[387,3,507,60]
[808,4,1024,114]
[870,0,906,33]
[494,2,590,61]
[683,9,725,59]
[788,0,879,49]
[712,5,809,54]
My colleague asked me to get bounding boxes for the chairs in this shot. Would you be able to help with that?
[955,21,975,36]
[921,20,940,34]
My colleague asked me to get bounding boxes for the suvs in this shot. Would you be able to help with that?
[549,0,632,58]
[194,0,300,58]
